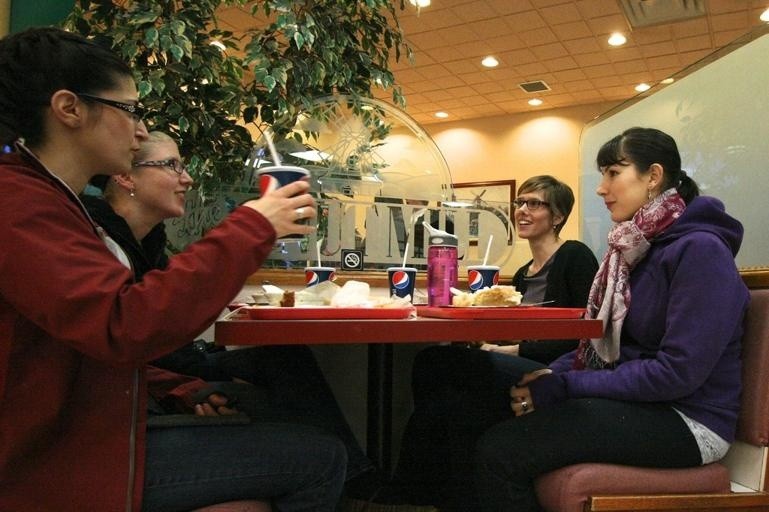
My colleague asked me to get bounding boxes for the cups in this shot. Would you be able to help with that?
[466,265,501,294]
[387,266,418,304]
[253,165,312,243]
[304,266,337,289]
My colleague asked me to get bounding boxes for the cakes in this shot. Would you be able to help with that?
[472,284,525,308]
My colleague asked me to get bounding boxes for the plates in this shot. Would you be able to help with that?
[250,292,310,305]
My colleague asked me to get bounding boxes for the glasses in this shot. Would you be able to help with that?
[512,198,550,211]
[45,92,149,122]
[132,158,188,173]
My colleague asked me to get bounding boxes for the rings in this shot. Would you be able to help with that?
[521,401,528,411]
[294,208,305,219]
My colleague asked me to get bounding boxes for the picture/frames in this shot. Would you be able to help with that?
[441,179,516,246]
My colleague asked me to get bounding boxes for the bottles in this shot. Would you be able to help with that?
[421,221,460,307]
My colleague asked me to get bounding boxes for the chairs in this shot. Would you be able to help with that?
[534,270,768,512]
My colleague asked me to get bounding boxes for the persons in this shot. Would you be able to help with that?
[91,131,412,503]
[466,126,750,512]
[1,27,348,511]
[404,175,600,507]
